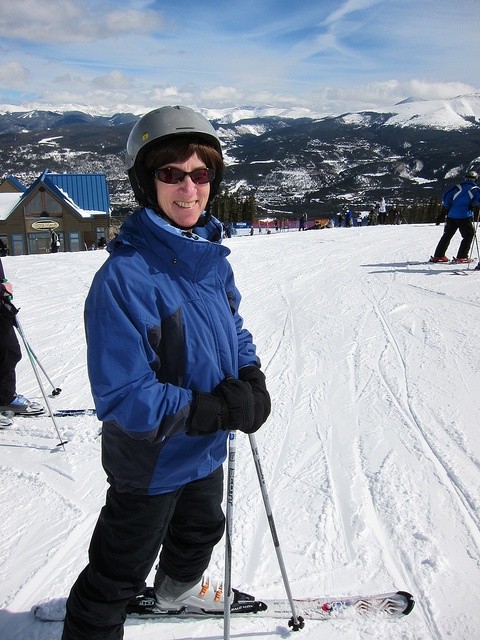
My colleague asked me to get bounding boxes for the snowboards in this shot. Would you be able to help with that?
[453,269,480,275]
[407,258,476,265]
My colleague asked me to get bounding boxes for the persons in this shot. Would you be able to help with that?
[298,215,306,230]
[336,212,344,227]
[1,255,48,425]
[61,104,272,637]
[326,217,335,228]
[346,215,352,227]
[434,170,480,265]
[48,228,60,252]
[356,212,363,227]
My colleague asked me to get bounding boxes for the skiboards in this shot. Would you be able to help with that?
[0,408,103,451]
[30,591,415,640]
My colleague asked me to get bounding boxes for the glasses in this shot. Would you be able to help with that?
[154,166,218,185]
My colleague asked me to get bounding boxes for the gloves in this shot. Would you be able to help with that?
[239,365,271,434]
[184,374,255,437]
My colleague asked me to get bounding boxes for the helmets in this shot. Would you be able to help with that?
[122,105,223,172]
[464,171,479,179]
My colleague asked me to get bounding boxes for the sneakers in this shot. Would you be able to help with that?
[433,256,448,262]
[0,394,31,411]
[0,413,14,429]
[153,572,235,610]
[456,257,470,263]
